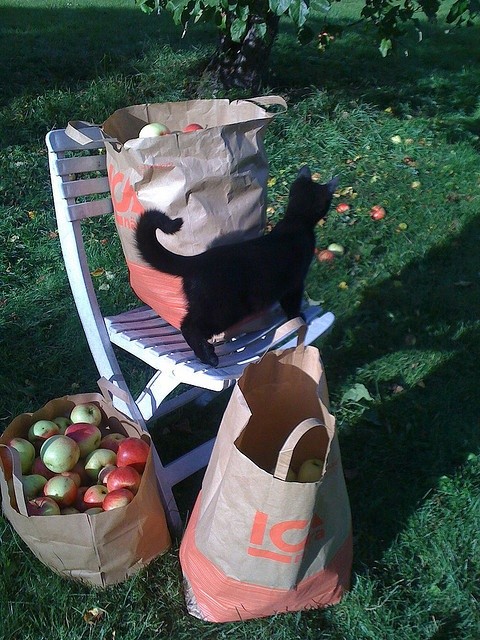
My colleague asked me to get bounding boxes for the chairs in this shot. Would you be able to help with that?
[45,122,339,542]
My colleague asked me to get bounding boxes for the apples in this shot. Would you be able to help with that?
[25,497,60,515]
[52,416,70,435]
[83,484,108,508]
[2,437,36,475]
[43,474,77,507]
[83,448,116,481]
[39,435,80,474]
[64,422,101,457]
[287,468,295,481]
[69,402,102,426]
[297,459,323,483]
[70,459,87,482]
[117,437,151,474]
[97,465,117,484]
[102,489,134,511]
[106,467,141,496]
[20,474,45,496]
[31,456,55,480]
[100,433,125,453]
[28,420,59,451]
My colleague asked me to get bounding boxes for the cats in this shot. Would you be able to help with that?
[133,166,343,364]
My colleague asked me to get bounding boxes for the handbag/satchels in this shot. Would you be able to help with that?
[1,376,171,591]
[179,317,354,623]
[64,96,287,344]
